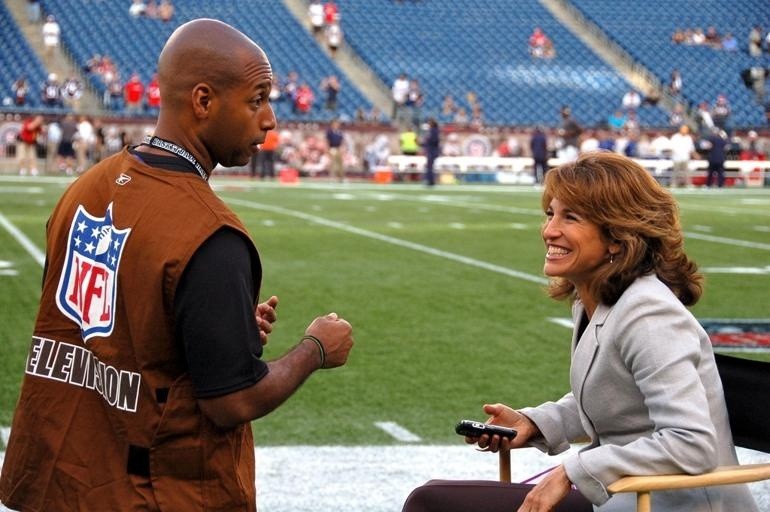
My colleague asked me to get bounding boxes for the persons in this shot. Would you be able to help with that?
[1,18,351,512]
[401,151,761,512]
[3,0,770,187]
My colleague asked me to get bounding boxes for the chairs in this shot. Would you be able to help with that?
[562,1,770,130]
[499,433,770,512]
[305,0,687,130]
[32,0,393,129]
[0,0,76,120]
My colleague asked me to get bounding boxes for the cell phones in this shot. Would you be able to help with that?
[455,420,517,442]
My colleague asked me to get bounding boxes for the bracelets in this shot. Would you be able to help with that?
[302,335,326,368]
